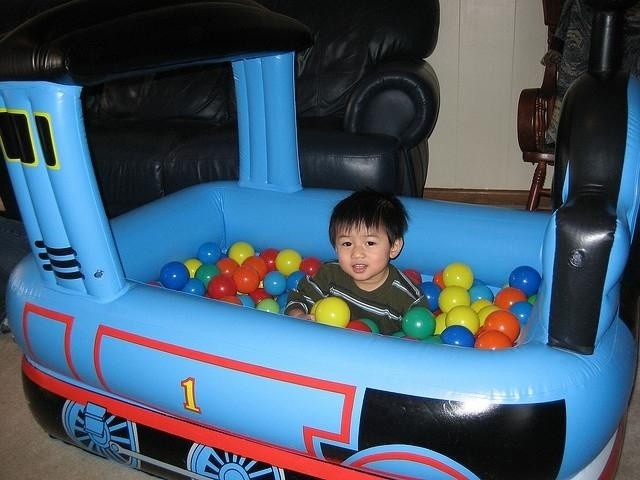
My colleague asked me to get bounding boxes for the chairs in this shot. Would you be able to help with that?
[517,0,640,211]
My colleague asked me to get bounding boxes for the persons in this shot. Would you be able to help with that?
[283,185,429,336]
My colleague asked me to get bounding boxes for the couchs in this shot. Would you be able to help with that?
[0,0,440,196]
